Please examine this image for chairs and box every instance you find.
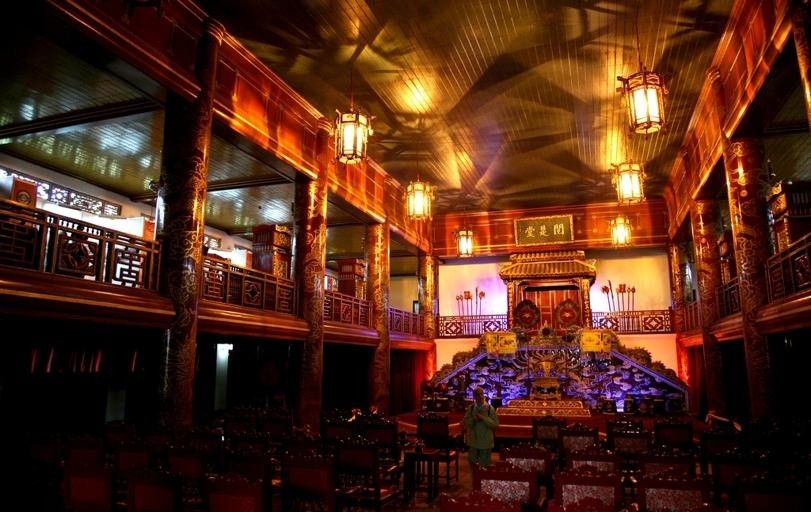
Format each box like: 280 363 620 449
72 404 805 511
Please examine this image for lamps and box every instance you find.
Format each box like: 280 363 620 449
608 1 671 249
332 62 478 260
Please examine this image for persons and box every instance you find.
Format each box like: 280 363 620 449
462 387 499 472
